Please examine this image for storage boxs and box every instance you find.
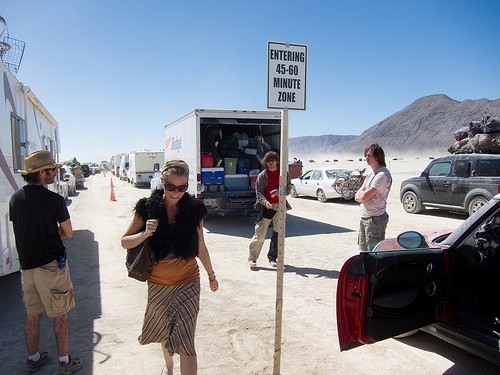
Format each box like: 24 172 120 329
249 174 257 190
201 168 224 185
224 174 249 191
245 148 257 155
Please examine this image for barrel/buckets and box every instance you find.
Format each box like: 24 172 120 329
202 152 214 168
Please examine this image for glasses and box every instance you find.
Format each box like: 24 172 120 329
164 179 188 192
44 168 56 172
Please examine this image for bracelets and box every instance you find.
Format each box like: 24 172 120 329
209 276 215 281
208 271 215 275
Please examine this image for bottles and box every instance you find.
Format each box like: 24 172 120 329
58 256 66 269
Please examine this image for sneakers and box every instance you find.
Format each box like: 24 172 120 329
24 350 50 374
56 355 83 375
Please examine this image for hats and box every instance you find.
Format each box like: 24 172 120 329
17 149 63 175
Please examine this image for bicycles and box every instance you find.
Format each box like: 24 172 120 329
334 168 367 199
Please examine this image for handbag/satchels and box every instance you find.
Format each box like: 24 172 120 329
125 198 156 282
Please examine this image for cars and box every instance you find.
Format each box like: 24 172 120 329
289 169 355 203
81 162 100 177
60 164 76 195
69 166 86 190
400 154 500 217
58 170 70 203
335 193 500 375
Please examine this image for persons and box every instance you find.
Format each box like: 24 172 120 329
248 151 292 267
355 143 392 252
120 159 218 375
8 149 84 375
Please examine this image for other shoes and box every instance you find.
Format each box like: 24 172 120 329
269 260 278 266
249 259 256 266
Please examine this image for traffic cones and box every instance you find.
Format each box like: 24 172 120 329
110 178 118 201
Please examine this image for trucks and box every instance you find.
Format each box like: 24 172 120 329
0 57 62 278
125 150 164 188
100 153 126 177
150 108 281 219
119 155 129 182
